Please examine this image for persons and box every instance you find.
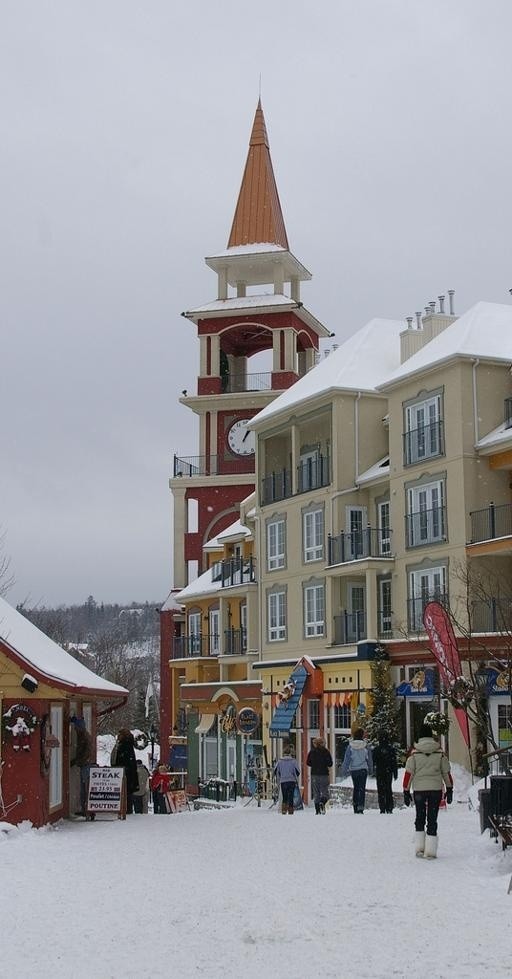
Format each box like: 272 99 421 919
338 728 373 816
402 722 455 862
67 716 97 817
372 729 399 815
305 735 334 815
405 734 447 812
272 745 301 816
108 727 139 813
132 758 176 814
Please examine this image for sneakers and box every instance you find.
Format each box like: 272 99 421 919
75 809 96 817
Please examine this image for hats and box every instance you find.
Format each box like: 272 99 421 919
74 719 85 728
119 728 130 738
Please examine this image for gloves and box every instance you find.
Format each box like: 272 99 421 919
444 788 454 804
403 790 413 807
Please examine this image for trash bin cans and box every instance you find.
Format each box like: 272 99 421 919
204 778 227 801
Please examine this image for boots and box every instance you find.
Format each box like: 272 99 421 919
413 831 438 860
281 797 394 815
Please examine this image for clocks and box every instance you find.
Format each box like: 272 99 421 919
224 416 256 459
